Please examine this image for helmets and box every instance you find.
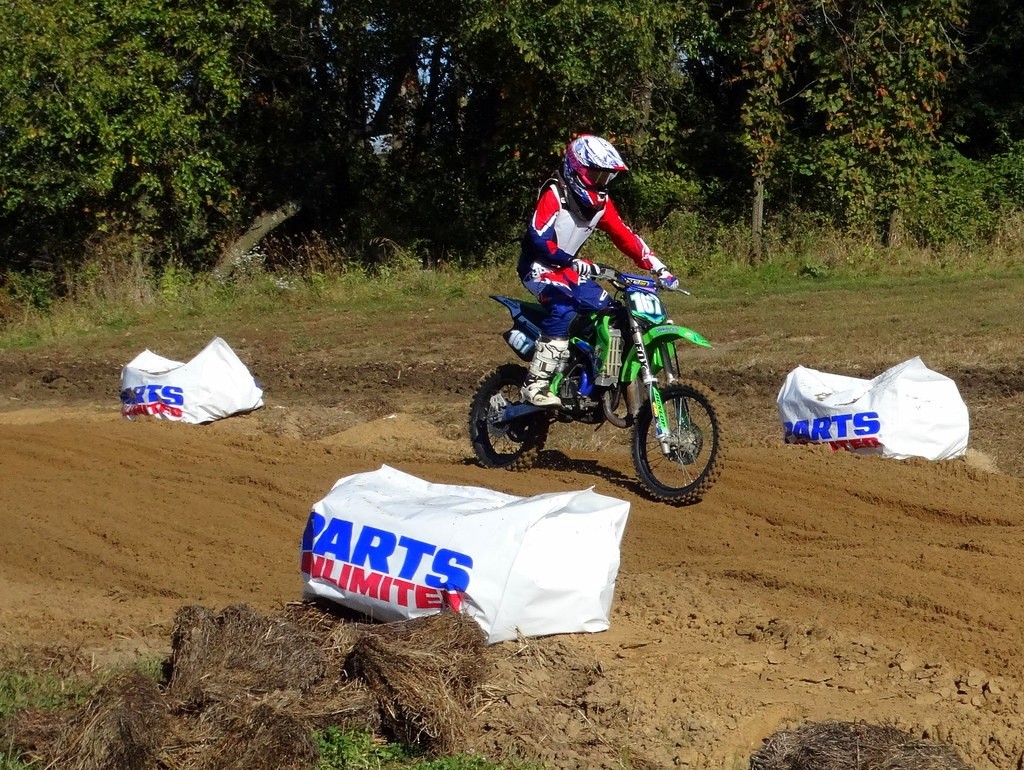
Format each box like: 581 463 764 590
559 135 629 210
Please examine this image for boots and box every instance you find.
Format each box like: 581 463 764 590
520 336 569 407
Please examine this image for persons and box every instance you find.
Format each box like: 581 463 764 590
514 136 680 407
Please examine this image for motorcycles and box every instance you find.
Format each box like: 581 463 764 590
467 262 729 506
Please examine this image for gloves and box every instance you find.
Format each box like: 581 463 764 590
572 259 600 277
657 268 679 291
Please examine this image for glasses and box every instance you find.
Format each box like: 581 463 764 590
580 166 619 189
